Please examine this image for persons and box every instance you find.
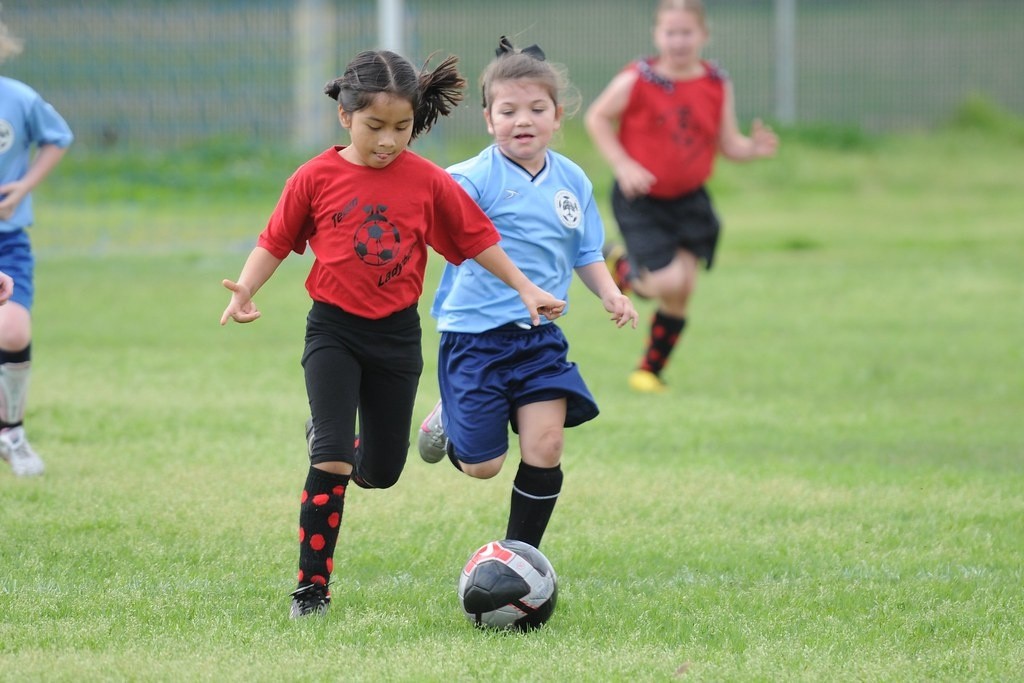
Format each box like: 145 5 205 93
0 25 73 478
419 36 639 549
583 0 779 394
220 51 566 622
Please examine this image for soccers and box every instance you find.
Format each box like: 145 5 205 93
456 538 560 637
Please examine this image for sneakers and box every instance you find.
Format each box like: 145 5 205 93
288 587 333 623
1 426 43 477
417 399 449 464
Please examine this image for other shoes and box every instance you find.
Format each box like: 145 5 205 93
627 371 675 395
601 237 627 297
